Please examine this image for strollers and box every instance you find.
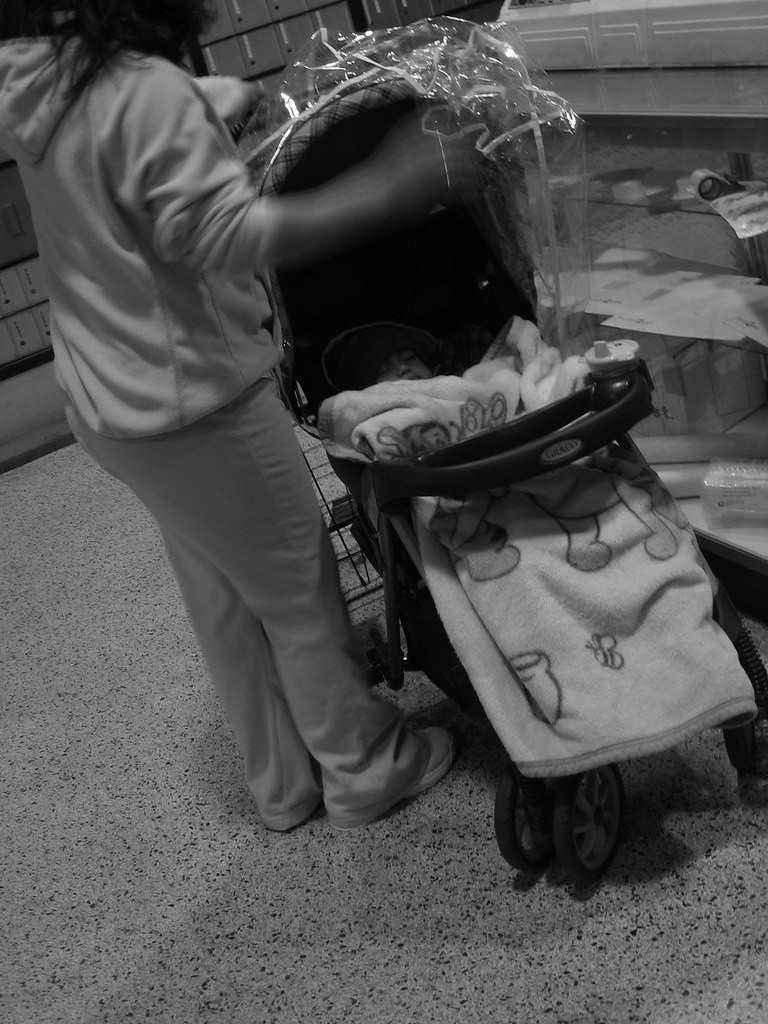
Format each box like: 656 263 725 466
246 73 768 879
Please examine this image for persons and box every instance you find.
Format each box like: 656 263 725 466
0 1 458 830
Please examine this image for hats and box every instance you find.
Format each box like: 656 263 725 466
322 317 438 392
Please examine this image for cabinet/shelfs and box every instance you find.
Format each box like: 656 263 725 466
538 171 768 631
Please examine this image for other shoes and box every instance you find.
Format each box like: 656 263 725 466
327 726 455 831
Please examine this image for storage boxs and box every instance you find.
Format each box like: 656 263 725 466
15 256 49 308
0 319 18 365
198 0 309 46
31 302 53 348
310 1 355 34
201 36 249 81
236 25 285 77
0 266 29 318
273 14 319 65
6 310 42 358
